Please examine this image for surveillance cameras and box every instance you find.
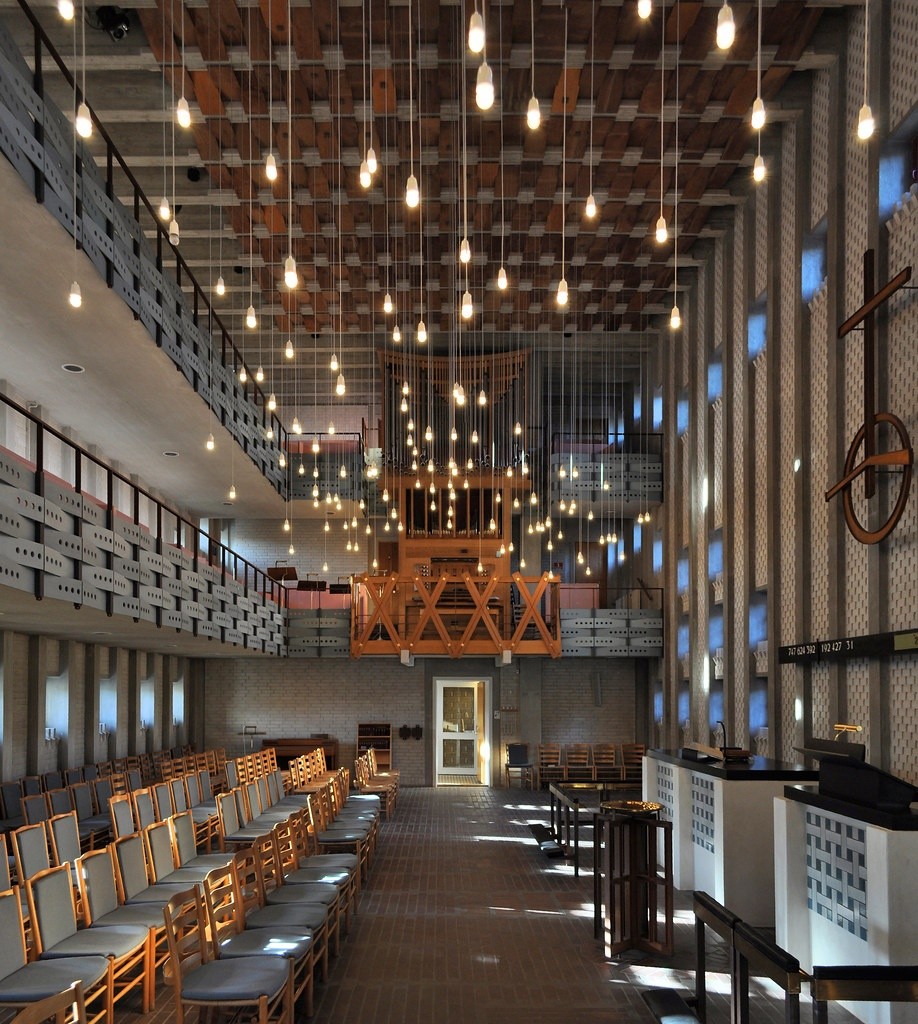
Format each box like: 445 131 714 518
95 6 127 42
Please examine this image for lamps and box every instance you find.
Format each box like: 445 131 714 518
56 0 874 580
834 724 863 742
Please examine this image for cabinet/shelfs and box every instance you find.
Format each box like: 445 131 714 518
356 722 392 771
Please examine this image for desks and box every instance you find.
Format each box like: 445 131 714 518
260 740 339 771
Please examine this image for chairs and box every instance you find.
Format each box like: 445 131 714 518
504 738 646 793
0 744 400 1024
